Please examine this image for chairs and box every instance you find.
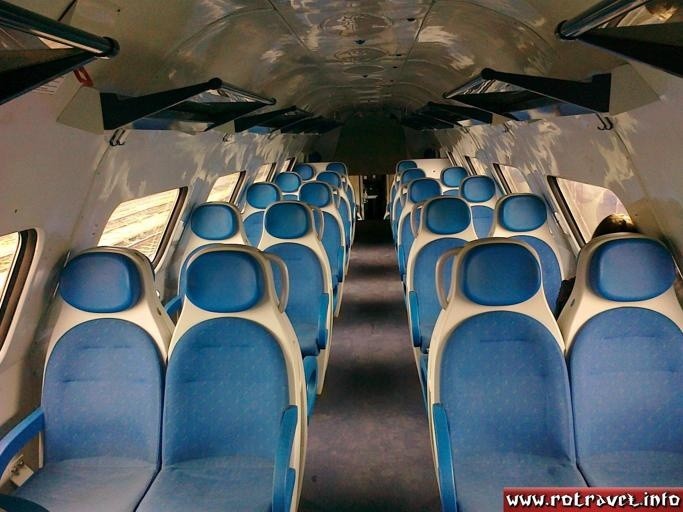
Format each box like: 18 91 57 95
0 158 683 512
